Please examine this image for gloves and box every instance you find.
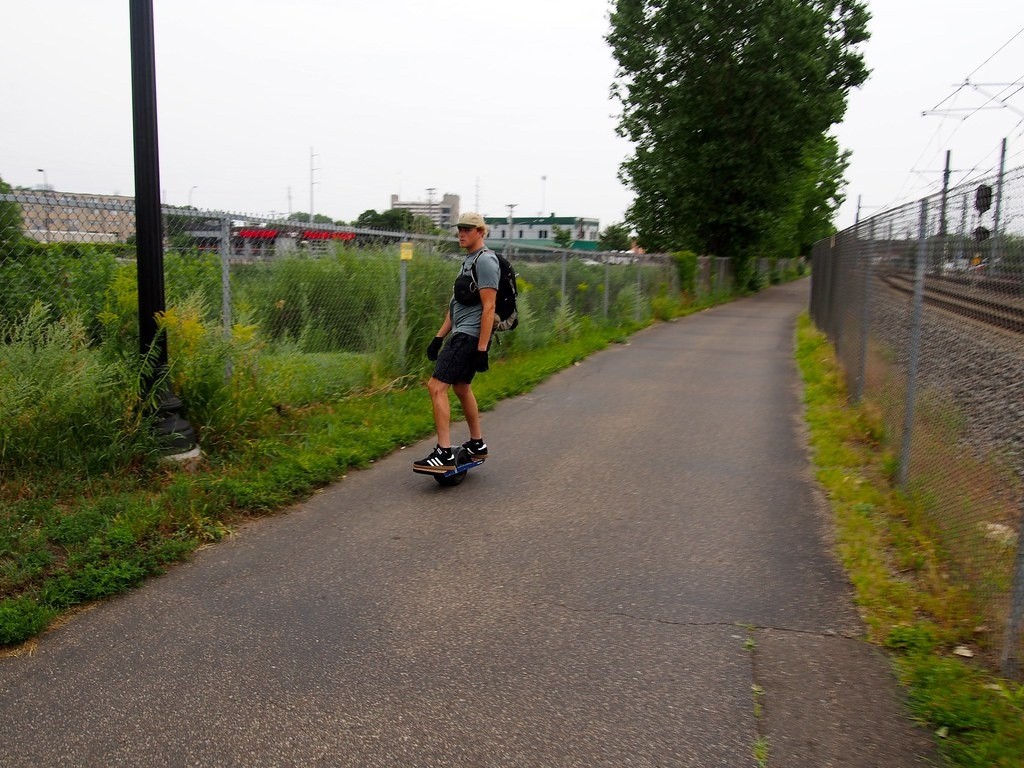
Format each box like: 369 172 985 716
472 350 489 372
427 337 443 360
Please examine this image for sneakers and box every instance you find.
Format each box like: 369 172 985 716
462 441 488 458
413 444 456 471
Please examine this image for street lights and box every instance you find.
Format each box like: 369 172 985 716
505 203 518 261
188 185 199 208
38 168 48 196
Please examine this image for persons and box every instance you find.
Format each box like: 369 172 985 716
413 212 501 470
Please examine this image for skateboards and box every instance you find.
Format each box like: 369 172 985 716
434 445 485 486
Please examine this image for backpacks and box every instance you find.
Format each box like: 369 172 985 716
470 250 519 332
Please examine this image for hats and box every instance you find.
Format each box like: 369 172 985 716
450 212 485 228
